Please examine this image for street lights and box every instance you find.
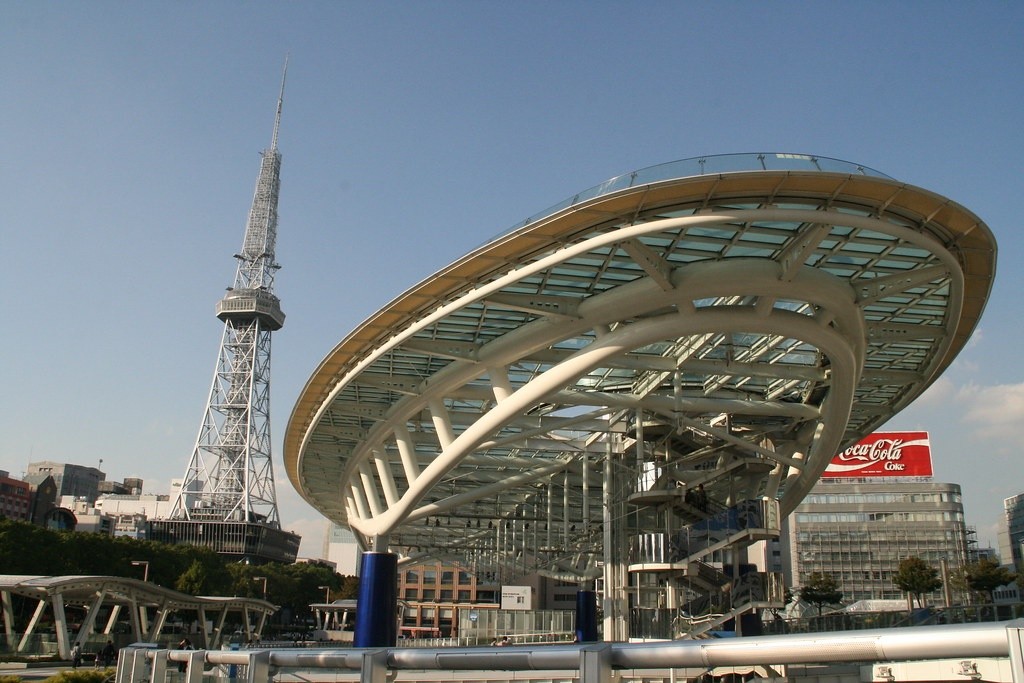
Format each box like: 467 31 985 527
318 586 329 604
254 576 266 601
131 561 149 582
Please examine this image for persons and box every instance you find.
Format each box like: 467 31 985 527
71 642 82 669
95 640 119 671
686 483 710 511
178 637 192 680
716 572 737 613
291 629 311 646
249 628 260 645
424 515 605 534
489 636 514 648
